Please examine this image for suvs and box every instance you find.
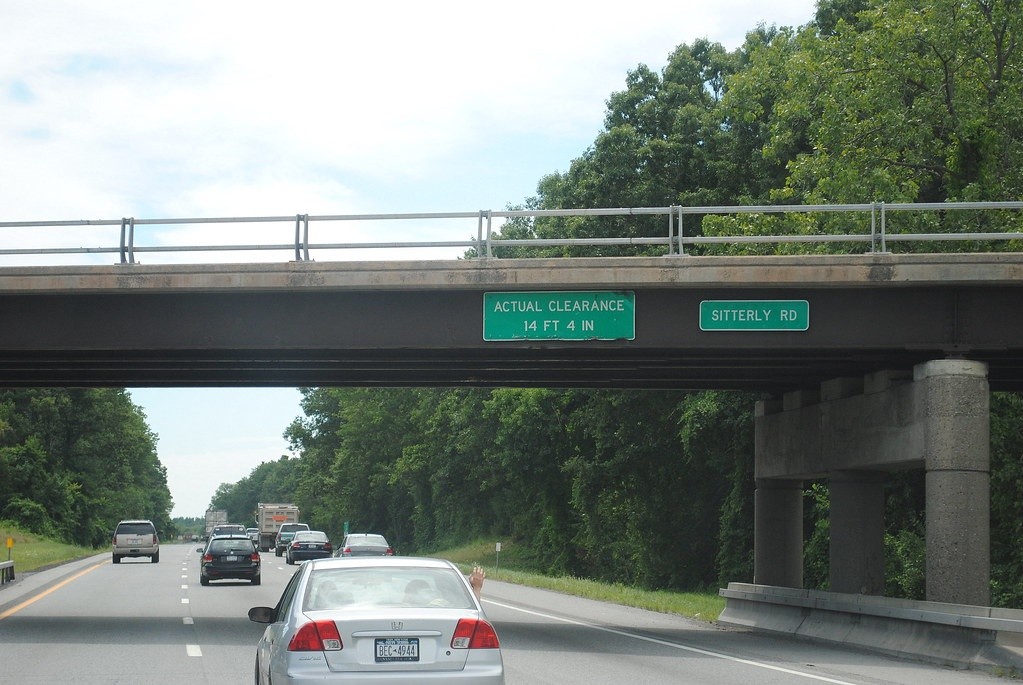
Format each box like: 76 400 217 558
196 532 262 586
112 519 160 563
202 524 254 546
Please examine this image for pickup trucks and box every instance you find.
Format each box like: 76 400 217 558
286 530 333 565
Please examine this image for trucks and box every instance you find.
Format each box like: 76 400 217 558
203 509 229 541
254 503 301 552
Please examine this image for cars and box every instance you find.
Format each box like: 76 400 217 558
249 554 506 685
334 533 395 557
246 528 259 544
271 522 312 556
